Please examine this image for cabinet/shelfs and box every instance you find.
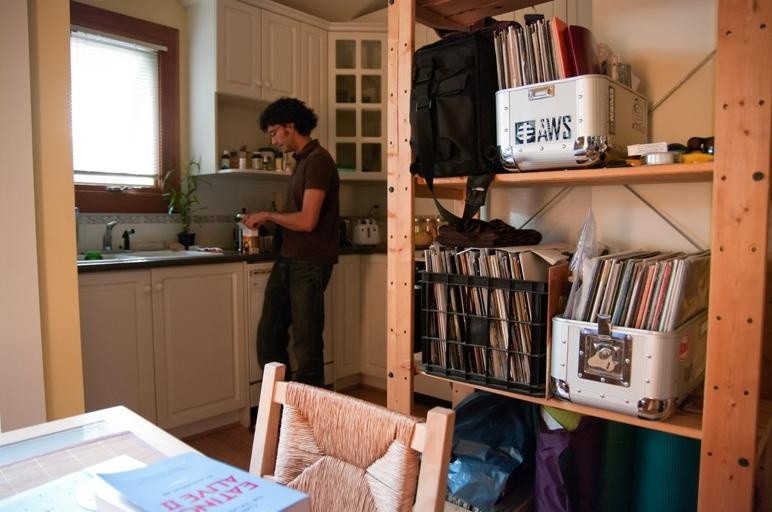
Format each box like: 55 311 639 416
214 92 293 174
216 1 300 101
329 29 387 174
76 265 244 424
387 0 772 512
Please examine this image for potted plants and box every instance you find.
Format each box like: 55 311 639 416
159 158 213 249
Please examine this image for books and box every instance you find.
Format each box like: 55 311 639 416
424 247 571 386
90 449 312 512
493 15 605 91
565 248 711 331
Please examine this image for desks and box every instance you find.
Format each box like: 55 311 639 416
0 402 212 512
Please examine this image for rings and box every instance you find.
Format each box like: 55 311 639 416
244 222 248 225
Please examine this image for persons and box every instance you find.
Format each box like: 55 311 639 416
236 94 342 439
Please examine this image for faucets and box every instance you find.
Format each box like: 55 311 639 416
122 228 135 250
103 221 118 250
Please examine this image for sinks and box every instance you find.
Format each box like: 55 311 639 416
77 253 137 264
124 250 224 258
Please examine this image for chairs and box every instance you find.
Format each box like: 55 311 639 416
243 361 457 511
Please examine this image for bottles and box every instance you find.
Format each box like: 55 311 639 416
221 146 284 171
237 208 260 257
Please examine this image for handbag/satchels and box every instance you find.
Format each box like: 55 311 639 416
407 12 523 179
